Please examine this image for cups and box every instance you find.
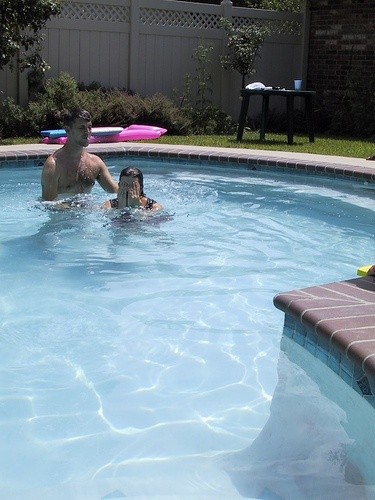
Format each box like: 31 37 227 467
294 80 302 90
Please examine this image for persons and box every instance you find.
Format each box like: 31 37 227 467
41 108 119 219
102 166 164 215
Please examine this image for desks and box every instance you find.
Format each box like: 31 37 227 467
236 89 315 146
